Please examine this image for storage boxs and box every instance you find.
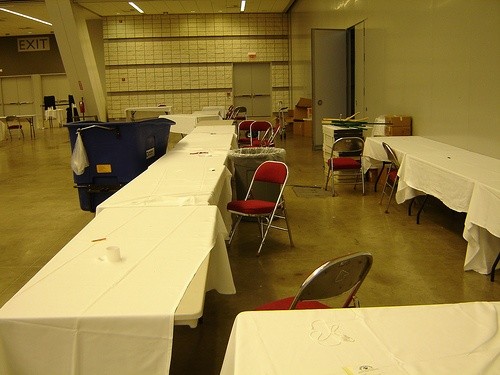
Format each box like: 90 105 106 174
373 116 413 135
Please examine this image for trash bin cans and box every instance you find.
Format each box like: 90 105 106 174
228 146 286 223
63 117 176 214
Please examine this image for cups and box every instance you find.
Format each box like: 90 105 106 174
106 246 120 262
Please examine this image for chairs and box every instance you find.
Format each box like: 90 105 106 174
230 109 240 120
254 253 373 310
6 116 25 140
227 161 295 255
250 121 274 147
234 106 247 120
379 142 426 215
225 105 234 120
325 137 366 197
237 120 255 148
261 125 281 146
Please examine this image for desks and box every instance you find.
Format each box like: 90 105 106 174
0 106 500 375
